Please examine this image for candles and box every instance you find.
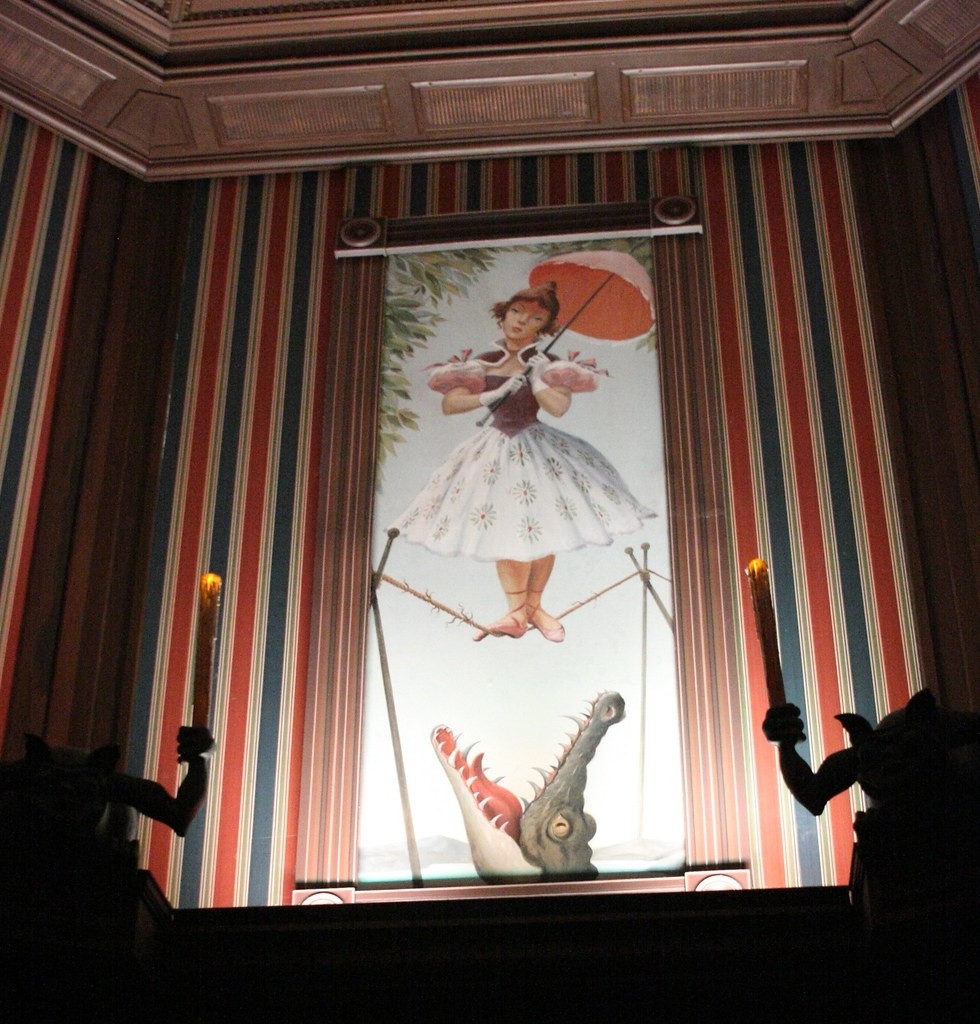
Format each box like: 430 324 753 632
192 573 222 728
745 559 788 707
